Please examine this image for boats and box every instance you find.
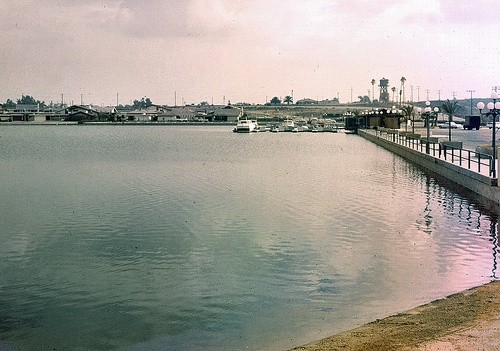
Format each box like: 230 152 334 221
232 116 340 133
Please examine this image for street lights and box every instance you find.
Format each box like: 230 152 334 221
416 100 439 154
400 76 406 117
476 91 500 178
391 87 396 113
371 78 376 111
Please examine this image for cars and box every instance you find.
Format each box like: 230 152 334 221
485 121 500 129
438 121 458 129
